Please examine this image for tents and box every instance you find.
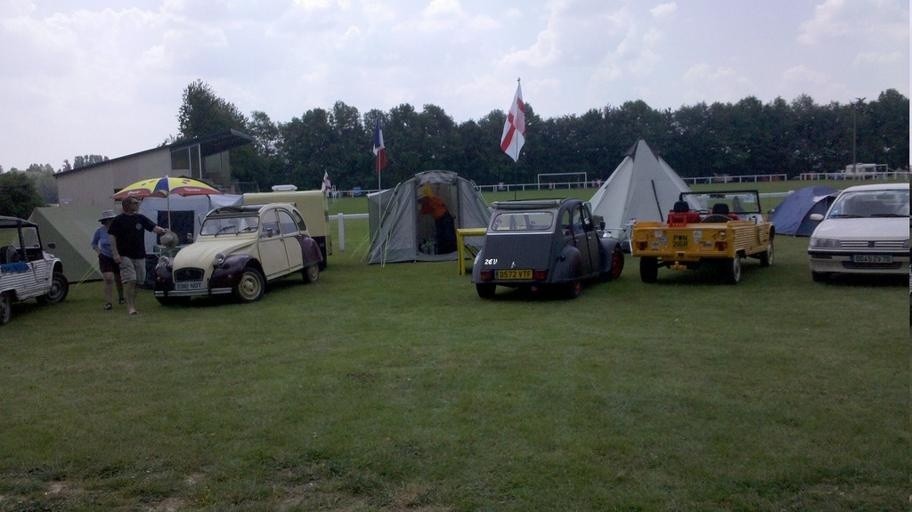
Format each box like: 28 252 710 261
767 184 843 237
18 206 105 283
572 136 704 254
366 170 492 265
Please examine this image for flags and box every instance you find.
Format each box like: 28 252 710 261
500 82 526 162
373 119 389 172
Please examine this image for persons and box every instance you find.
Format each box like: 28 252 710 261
90 210 126 311
108 196 168 315
416 197 457 254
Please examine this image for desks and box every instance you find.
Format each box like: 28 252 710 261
455 228 486 276
144 251 177 289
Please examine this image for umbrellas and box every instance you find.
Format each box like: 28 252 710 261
110 175 222 230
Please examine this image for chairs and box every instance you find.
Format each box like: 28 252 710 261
702 214 732 224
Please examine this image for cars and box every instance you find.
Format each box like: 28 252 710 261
152 199 325 311
704 173 735 185
350 186 363 199
805 181 912 290
467 196 628 304
755 162 910 183
496 182 507 192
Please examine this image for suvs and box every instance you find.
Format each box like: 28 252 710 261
630 182 779 289
1 213 71 331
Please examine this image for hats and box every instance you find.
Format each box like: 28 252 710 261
96 210 118 222
